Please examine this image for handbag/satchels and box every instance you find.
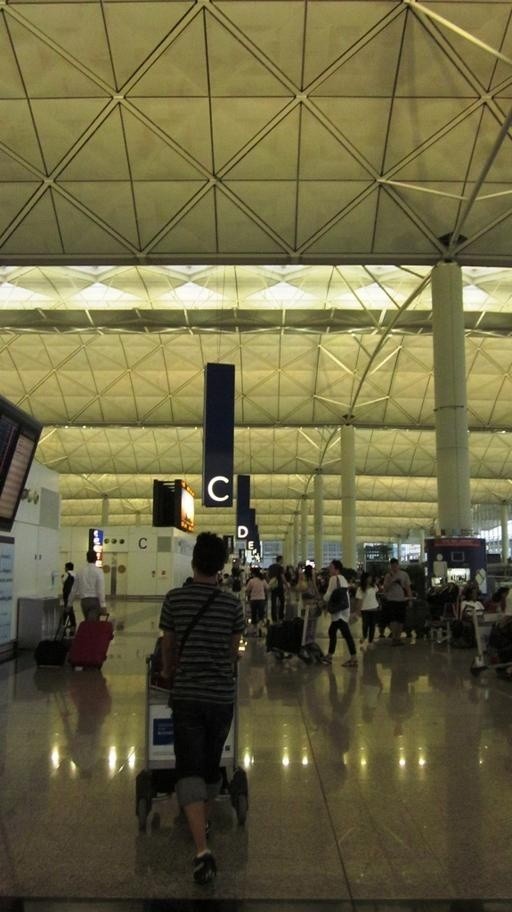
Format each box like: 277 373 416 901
326 587 349 613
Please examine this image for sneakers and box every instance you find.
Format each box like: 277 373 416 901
192 851 218 885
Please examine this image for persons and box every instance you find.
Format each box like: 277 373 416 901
65 549 107 618
159 530 245 885
61 562 77 636
231 553 512 680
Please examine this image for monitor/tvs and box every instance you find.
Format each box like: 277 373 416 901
448 568 470 584
0 395 44 532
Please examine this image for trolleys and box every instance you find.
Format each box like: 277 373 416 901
136 637 249 830
266 603 324 663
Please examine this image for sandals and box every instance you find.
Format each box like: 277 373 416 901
318 656 333 664
341 659 358 668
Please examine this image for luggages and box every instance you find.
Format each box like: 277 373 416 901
34 612 69 668
67 613 114 671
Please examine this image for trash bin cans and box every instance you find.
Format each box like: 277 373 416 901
17 596 60 651
